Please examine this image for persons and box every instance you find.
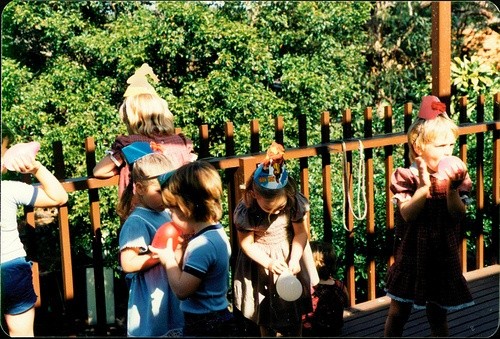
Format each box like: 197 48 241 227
381 115 477 338
92 91 199 218
147 158 231 338
1 122 69 337
120 151 185 338
230 162 321 338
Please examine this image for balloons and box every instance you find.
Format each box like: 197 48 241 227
275 271 303 302
151 221 181 251
438 156 466 179
